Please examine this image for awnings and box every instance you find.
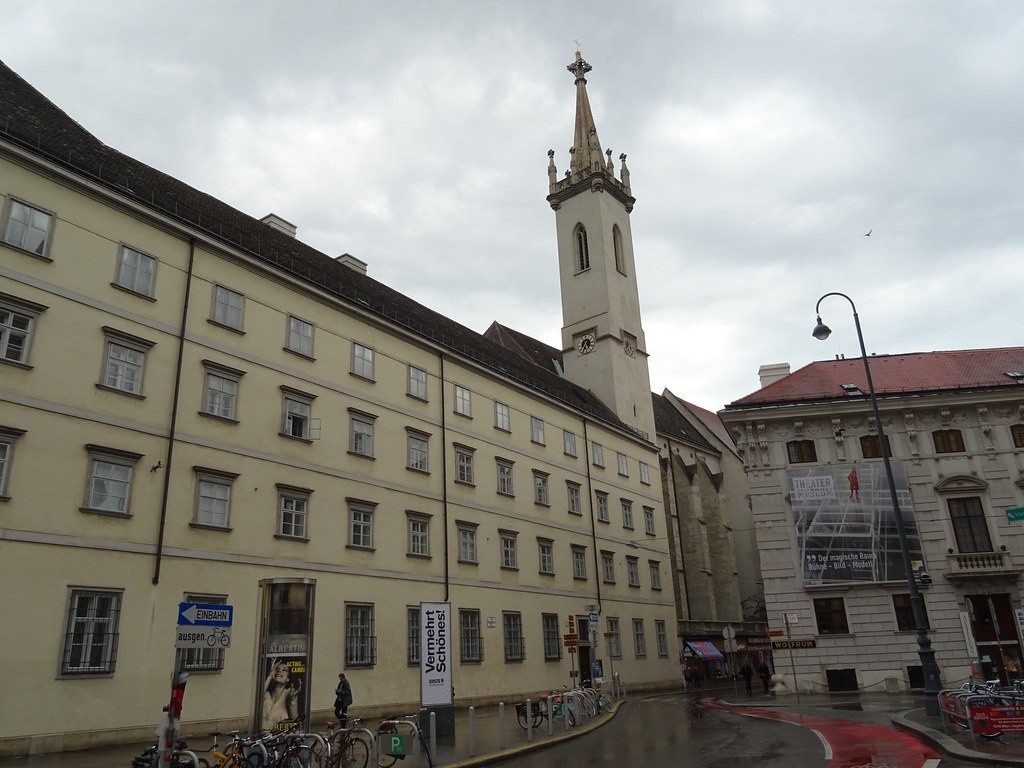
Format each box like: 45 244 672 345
685 639 724 661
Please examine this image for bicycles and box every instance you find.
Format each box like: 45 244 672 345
514 678 619 729
369 706 433 768
128 705 370 768
948 678 1024 739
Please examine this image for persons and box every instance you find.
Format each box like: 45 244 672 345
334 673 353 730
759 661 770 695
684 665 701 689
738 662 756 695
261 657 302 731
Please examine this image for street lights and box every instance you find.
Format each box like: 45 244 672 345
809 292 948 716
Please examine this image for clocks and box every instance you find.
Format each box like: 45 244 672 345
622 336 634 355
577 334 595 355
